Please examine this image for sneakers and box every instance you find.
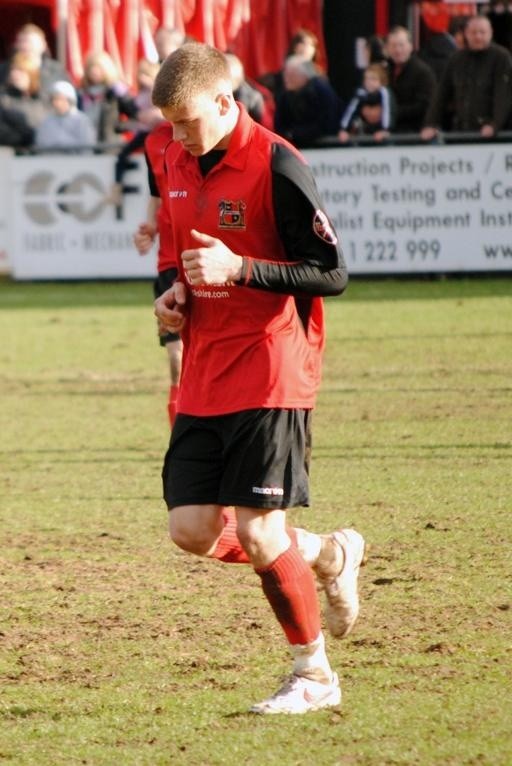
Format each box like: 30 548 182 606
249 673 342 716
325 525 366 640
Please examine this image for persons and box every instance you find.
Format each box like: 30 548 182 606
153 44 363 714
438 13 512 145
133 120 183 429
220 29 439 149
0 24 165 149
